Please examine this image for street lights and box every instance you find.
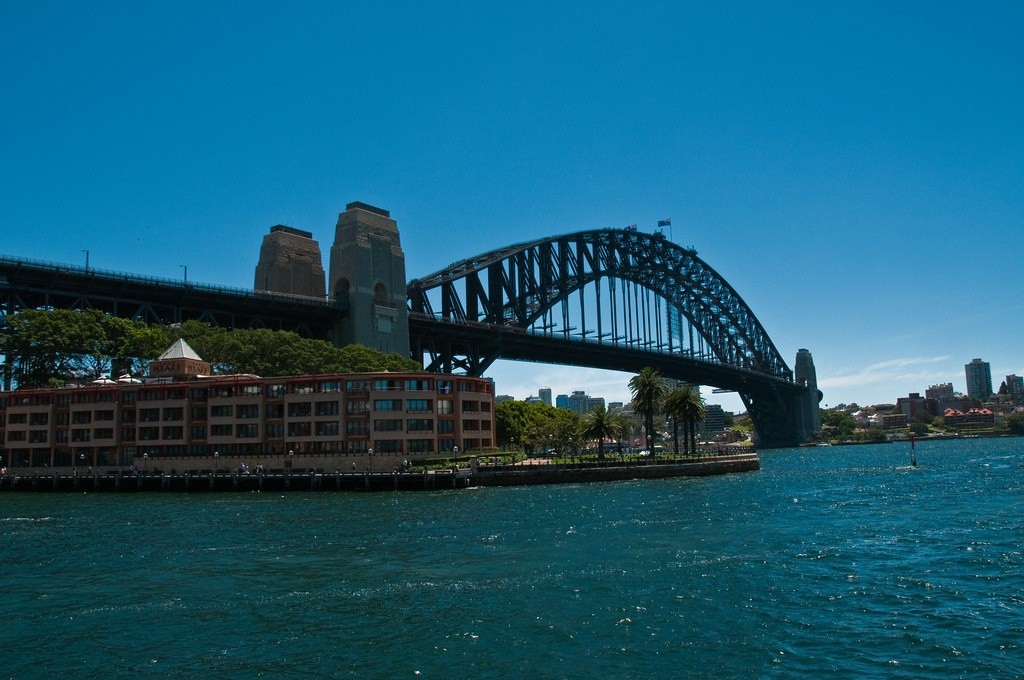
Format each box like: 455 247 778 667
453 445 459 466
214 451 219 474
697 434 701 454
80 454 84 476
511 436 516 472
143 453 148 474
368 448 373 473
179 264 187 282
289 450 293 475
82 249 90 273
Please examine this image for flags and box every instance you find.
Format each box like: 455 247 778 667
658 218 670 226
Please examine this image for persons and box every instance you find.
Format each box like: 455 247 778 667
402 458 412 472
129 464 138 476
352 460 357 472
0 466 8 477
241 463 263 476
74 466 93 477
